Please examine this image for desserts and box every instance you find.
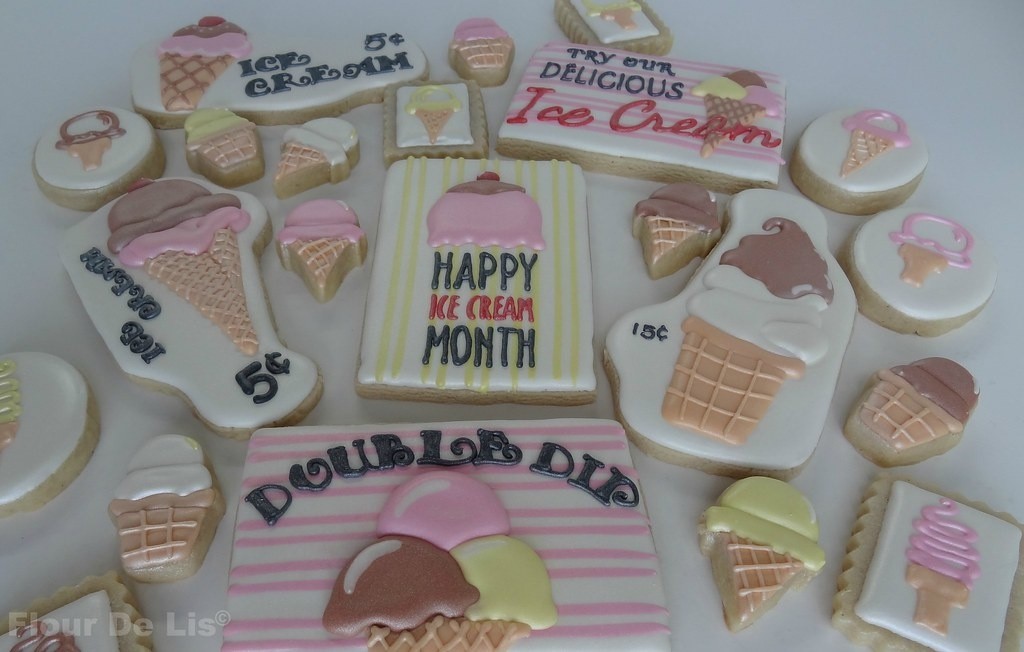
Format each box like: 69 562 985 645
553 0 673 56
184 108 264 187
274 117 359 198
60 178 324 440
275 199 367 304
497 42 787 193
221 418 673 652
603 187 858 482
790 107 929 214
842 209 997 336
32 106 166 212
106 433 225 582
696 476 825 634
0 352 100 518
449 17 515 86
383 80 488 165
0 572 153 652
843 354 978 467
355 155 597 405
632 181 721 278
130 16 430 131
832 468 1024 652
